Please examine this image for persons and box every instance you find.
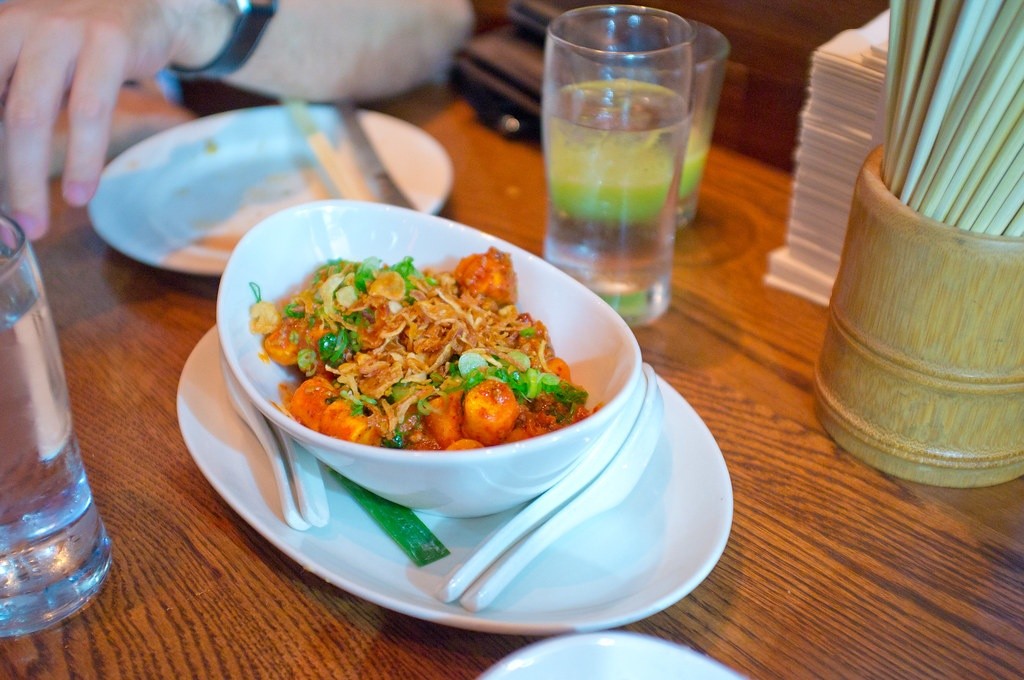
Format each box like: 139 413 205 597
0 0 476 250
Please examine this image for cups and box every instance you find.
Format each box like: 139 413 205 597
540 5 731 332
0 211 111 639
812 144 1024 488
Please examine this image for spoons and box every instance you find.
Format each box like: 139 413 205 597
219 341 329 530
439 361 663 612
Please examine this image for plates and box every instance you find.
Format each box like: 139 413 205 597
176 318 734 680
89 99 455 277
473 630 745 680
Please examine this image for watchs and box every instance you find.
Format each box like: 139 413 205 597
170 0 279 79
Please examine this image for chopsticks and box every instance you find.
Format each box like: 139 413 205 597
884 0 1024 236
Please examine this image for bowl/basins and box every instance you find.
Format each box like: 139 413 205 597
217 199 643 518
